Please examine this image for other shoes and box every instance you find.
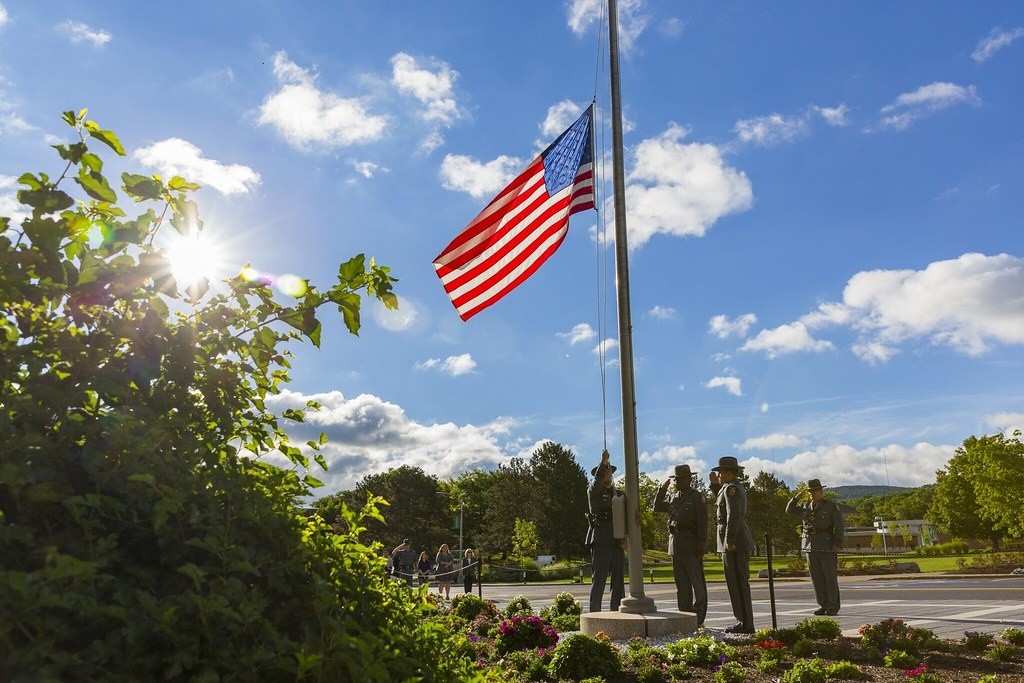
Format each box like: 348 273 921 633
826 609 838 615
814 607 826 615
725 623 755 634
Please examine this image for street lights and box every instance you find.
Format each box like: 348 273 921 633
435 490 464 561
875 516 887 556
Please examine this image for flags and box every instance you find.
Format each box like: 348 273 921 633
432 99 598 322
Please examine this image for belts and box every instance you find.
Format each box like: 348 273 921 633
670 523 697 531
803 528 831 535
716 517 725 524
401 564 414 570
590 512 611 521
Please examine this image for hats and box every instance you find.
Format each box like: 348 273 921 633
403 539 412 544
669 464 697 477
806 478 827 491
711 456 745 471
591 462 618 476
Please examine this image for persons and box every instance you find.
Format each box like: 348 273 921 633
654 465 709 627
584 449 628 612
369 539 417 587
417 551 431 585
785 480 844 616
436 544 454 600
462 549 477 594
709 456 755 633
